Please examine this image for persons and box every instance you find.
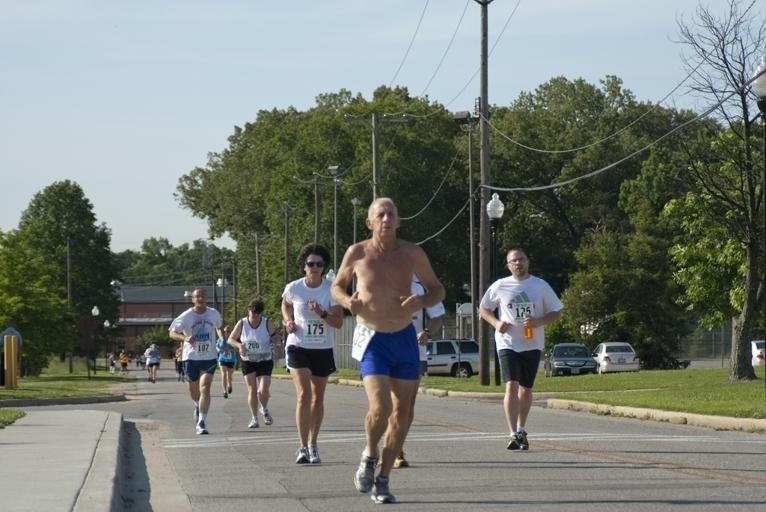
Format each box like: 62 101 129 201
168 288 225 435
109 342 184 382
281 243 343 463
0 325 23 386
329 198 447 504
479 247 564 449
228 298 283 428
377 274 446 468
216 324 237 398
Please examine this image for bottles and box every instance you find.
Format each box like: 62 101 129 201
522 311 534 341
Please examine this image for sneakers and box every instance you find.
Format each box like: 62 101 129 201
259 408 273 426
393 451 410 468
354 445 380 493
307 442 319 464
370 475 396 506
295 445 309 464
223 385 233 399
507 430 530 451
247 416 259 428
193 401 209 435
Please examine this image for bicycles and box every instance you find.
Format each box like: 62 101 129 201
639 353 691 370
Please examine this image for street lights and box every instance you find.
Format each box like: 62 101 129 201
349 195 360 294
215 277 228 324
486 191 507 386
103 319 110 371
90 306 101 375
109 278 123 361
184 289 191 308
450 107 493 341
328 161 340 275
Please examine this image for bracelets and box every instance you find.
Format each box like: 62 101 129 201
424 328 432 337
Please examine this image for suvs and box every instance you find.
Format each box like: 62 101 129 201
423 337 481 378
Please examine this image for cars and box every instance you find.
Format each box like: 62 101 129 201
592 342 639 373
543 343 596 377
750 340 766 366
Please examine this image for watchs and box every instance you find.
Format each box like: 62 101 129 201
321 309 328 318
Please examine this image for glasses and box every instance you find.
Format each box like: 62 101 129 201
304 261 324 268
